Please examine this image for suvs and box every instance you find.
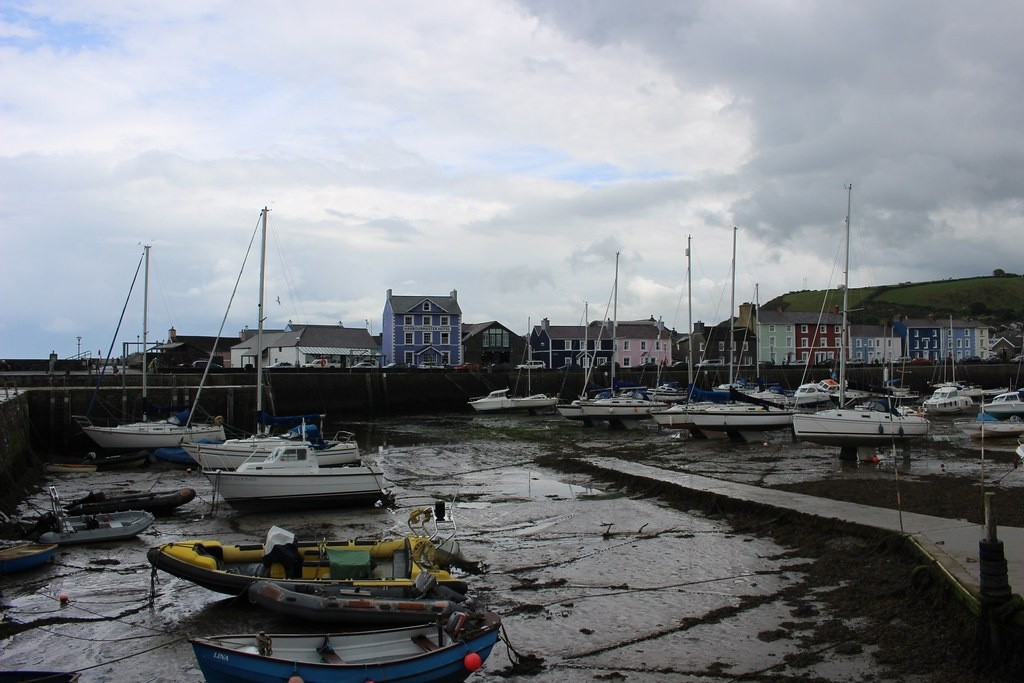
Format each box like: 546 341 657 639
514 360 547 370
892 357 911 363
847 357 865 365
418 362 445 369
193 361 223 369
695 359 723 368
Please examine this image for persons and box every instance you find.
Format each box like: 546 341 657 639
772 358 886 368
319 358 448 371
46 355 123 376
149 357 157 373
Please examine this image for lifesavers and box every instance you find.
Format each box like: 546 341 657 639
215 416 224 426
410 507 431 524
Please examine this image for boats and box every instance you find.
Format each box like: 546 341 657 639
147 497 501 683
923 314 1024 440
0 670 83 683
0 484 197 575
196 413 396 509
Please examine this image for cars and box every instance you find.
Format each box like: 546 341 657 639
453 362 479 370
381 362 408 369
556 364 580 371
758 360 775 368
345 362 380 369
264 361 296 368
640 363 656 368
1011 356 1024 363
912 358 930 365
788 360 806 366
818 358 836 367
959 355 981 363
669 361 687 368
594 361 620 368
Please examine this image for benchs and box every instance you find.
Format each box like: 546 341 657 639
315 635 346 664
109 521 123 528
411 634 440 652
62 521 75 533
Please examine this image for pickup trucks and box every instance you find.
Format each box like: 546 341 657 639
301 358 341 369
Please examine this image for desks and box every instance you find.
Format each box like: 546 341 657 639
340 588 371 596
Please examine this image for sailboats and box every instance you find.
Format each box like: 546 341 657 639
556 234 921 426
790 185 929 443
176 205 361 473
72 241 228 449
466 314 559 414
682 226 801 431
